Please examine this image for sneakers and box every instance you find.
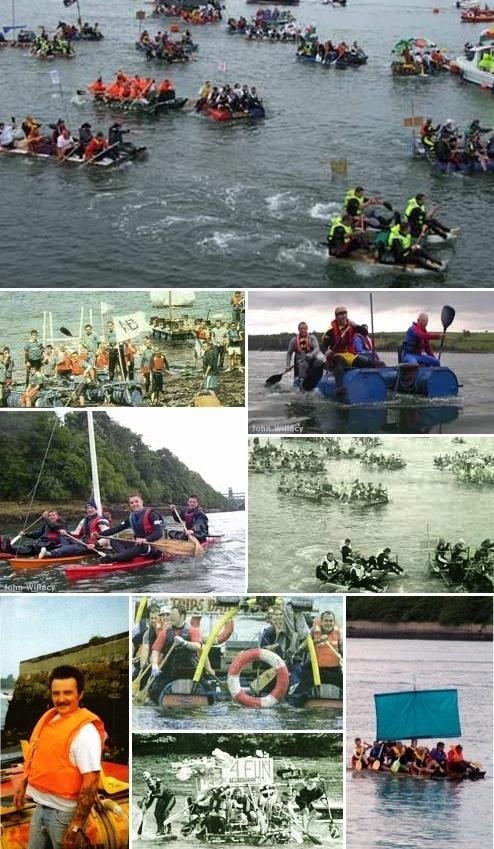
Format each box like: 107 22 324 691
0 535 5 552
39 547 47 558
5 537 12 552
335 387 345 398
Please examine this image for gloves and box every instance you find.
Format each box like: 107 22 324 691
149 667 162 679
174 636 187 647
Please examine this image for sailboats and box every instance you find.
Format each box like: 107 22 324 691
149 289 214 347
6 410 113 573
353 685 487 780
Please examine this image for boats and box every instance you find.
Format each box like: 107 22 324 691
420 138 494 175
328 219 462 276
285 405 460 432
179 750 305 846
294 363 465 404
0 552 29 558
94 90 190 114
8 365 143 407
0 746 129 849
63 535 221 581
132 597 343 713
198 104 265 121
248 437 494 593
0 139 149 168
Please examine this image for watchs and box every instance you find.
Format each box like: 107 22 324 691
70 824 82 833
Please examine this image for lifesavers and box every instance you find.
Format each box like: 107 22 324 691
227 648 288 708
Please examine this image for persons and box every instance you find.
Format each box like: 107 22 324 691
132 602 343 706
352 736 481 775
388 1 494 178
139 770 330 838
247 437 494 512
283 303 447 387
11 664 108 849
312 534 494 594
0 290 245 407
0 0 371 166
91 495 168 564
38 495 111 559
329 182 455 280
168 494 209 542
0 509 68 555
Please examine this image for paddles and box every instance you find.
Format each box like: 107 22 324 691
323 785 340 839
391 745 404 772
96 535 194 556
282 810 322 845
163 795 206 826
372 744 383 770
417 206 439 245
303 326 350 390
172 507 200 555
266 359 304 383
356 741 364 771
60 327 83 347
137 791 147 835
438 306 454 362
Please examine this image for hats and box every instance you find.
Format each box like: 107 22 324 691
157 606 171 615
85 498 96 509
335 306 346 316
